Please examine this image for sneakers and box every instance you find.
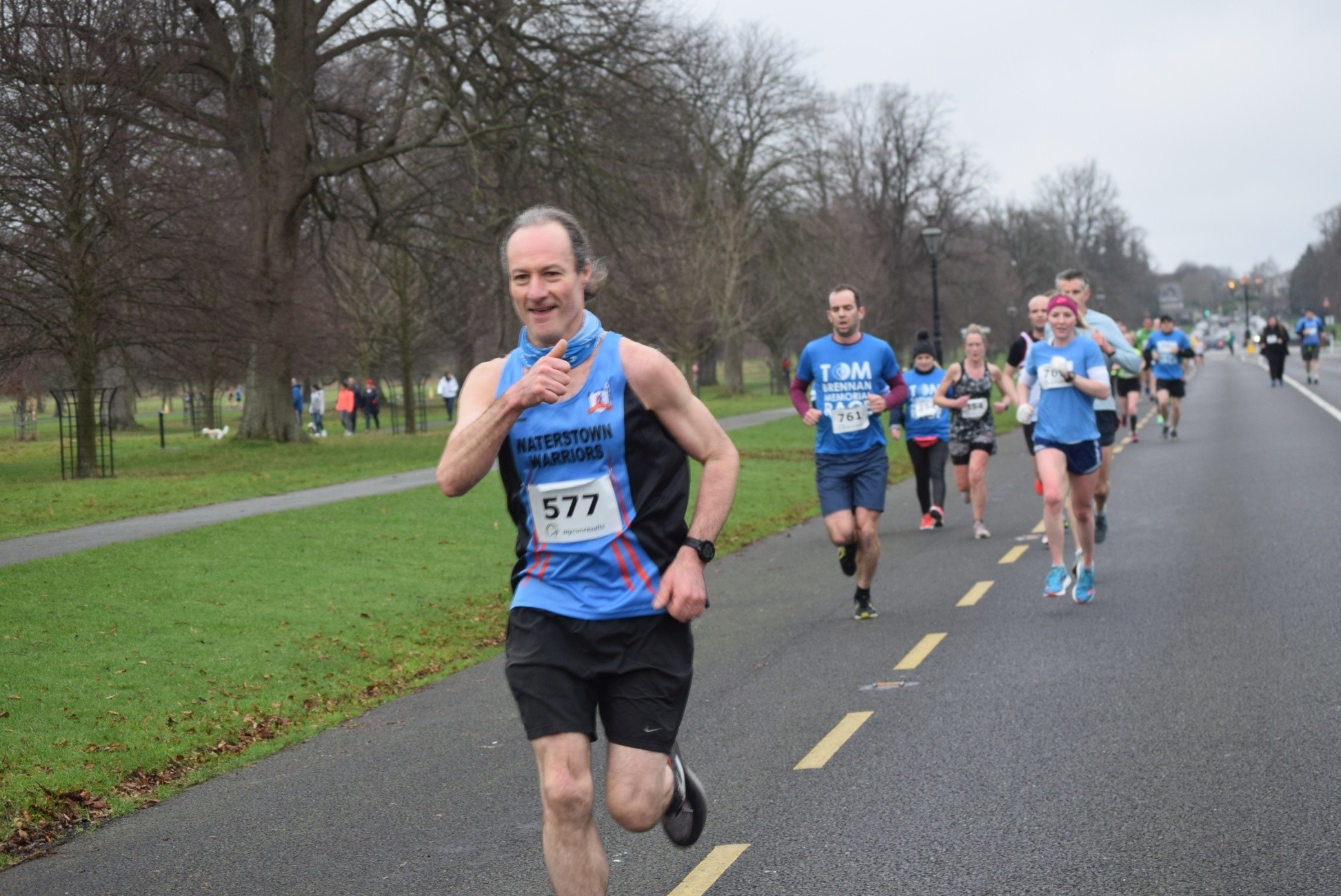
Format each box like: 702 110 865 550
973 521 991 539
1071 560 1096 602
662 741 708 848
920 514 935 530
837 541 858 577
1043 564 1071 597
930 505 944 527
963 491 971 502
853 593 877 619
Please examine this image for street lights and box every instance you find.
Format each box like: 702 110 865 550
1228 276 1265 343
1005 301 1018 344
921 215 943 370
1097 287 1105 313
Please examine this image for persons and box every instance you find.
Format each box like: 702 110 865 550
1295 309 1326 384
336 377 360 435
291 377 303 426
933 324 1011 539
437 371 459 421
889 330 953 530
783 356 790 386
692 362 699 382
308 384 327 437
360 380 380 432
791 284 909 620
1015 293 1111 603
1002 295 1069 529
1260 315 1289 387
1111 313 1204 443
227 385 242 410
1044 268 1142 577
1226 330 1234 354
436 205 741 896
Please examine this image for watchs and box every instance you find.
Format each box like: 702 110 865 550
1066 372 1075 382
682 536 715 562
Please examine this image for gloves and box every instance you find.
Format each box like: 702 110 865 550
1049 356 1074 376
1017 405 1035 424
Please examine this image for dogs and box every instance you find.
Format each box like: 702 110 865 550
202 426 229 440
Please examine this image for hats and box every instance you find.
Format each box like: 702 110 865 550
912 330 935 358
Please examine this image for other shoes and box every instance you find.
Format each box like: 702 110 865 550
1170 430 1178 438
1314 379 1318 384
1132 435 1137 442
1308 378 1311 383
1094 514 1107 543
1271 381 1276 386
1036 479 1044 495
1163 427 1169 433
1279 380 1283 386
